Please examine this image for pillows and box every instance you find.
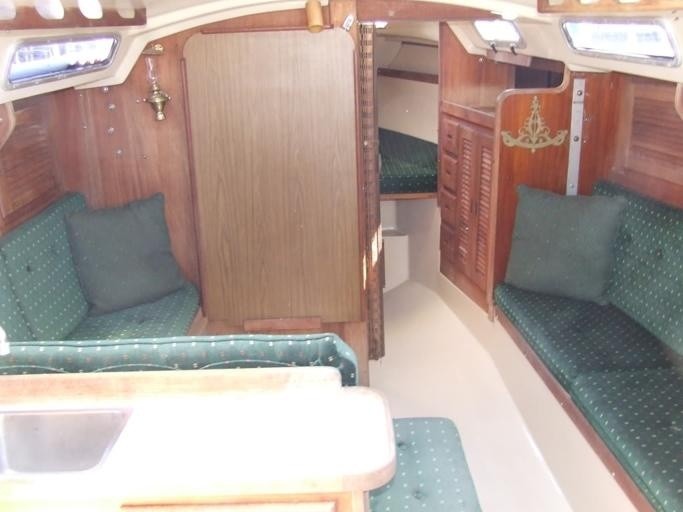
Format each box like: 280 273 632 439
58 191 190 317
502 182 628 308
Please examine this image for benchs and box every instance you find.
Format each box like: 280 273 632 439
0 191 359 382
490 178 682 512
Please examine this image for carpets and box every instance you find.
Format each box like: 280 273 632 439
369 413 483 512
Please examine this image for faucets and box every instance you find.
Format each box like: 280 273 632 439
0 325 11 357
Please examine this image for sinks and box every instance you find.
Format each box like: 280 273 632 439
1 403 138 476
